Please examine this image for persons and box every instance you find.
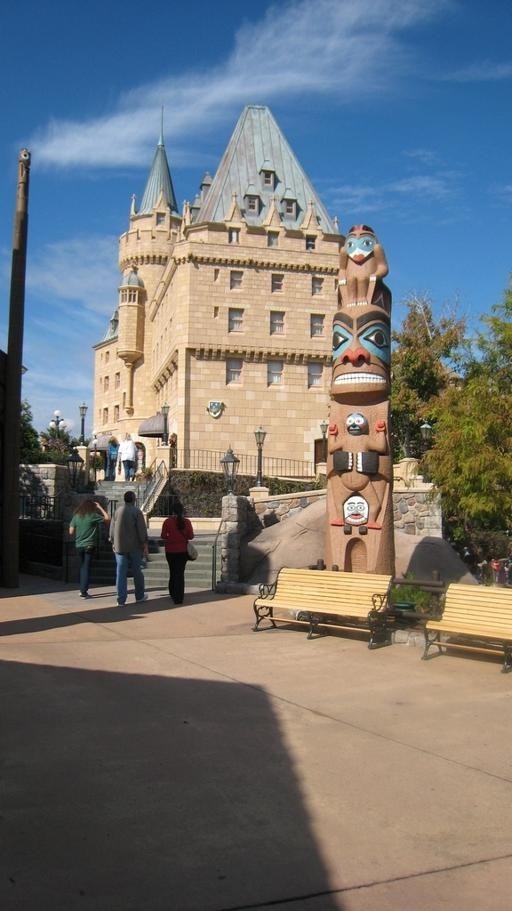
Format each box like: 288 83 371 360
104 436 120 481
457 544 512 586
108 490 149 607
118 432 138 482
160 503 194 604
68 498 111 599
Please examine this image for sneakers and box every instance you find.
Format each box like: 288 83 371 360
117 601 126 607
79 592 90 599
136 594 148 603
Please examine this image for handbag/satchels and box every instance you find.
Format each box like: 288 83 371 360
187 542 198 561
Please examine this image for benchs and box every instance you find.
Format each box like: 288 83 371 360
253 566 512 673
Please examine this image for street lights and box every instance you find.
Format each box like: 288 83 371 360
92 430 98 483
79 402 89 446
48 410 68 438
320 420 329 461
419 420 433 483
254 423 267 487
